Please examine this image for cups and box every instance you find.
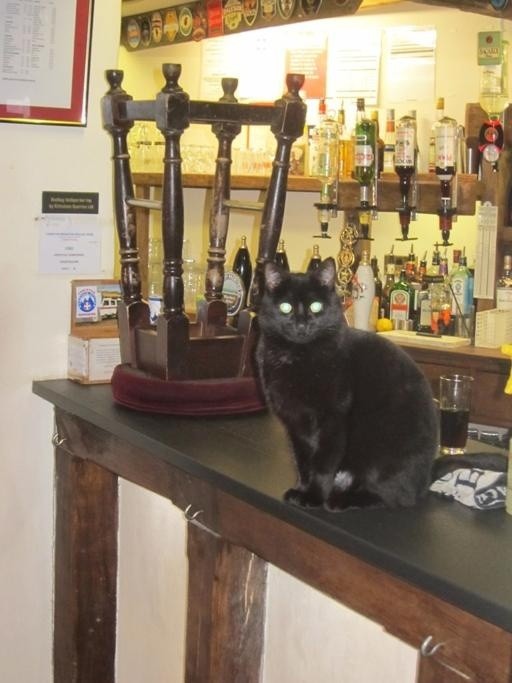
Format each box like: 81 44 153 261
438 373 476 456
455 314 475 338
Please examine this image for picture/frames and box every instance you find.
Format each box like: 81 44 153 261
0 0 95 126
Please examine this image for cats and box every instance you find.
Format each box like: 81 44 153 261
253 255 509 514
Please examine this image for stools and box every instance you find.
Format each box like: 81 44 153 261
102 60 307 420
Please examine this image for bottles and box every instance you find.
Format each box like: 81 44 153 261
309 93 460 209
479 38 512 124
351 249 475 331
495 250 512 311
